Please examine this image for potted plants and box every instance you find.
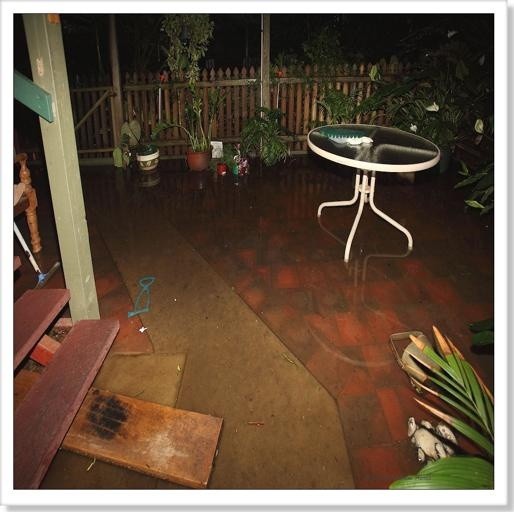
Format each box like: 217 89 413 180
386 84 463 177
110 113 161 171
240 104 294 181
151 85 234 175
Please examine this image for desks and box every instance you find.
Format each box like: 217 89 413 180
306 123 442 265
